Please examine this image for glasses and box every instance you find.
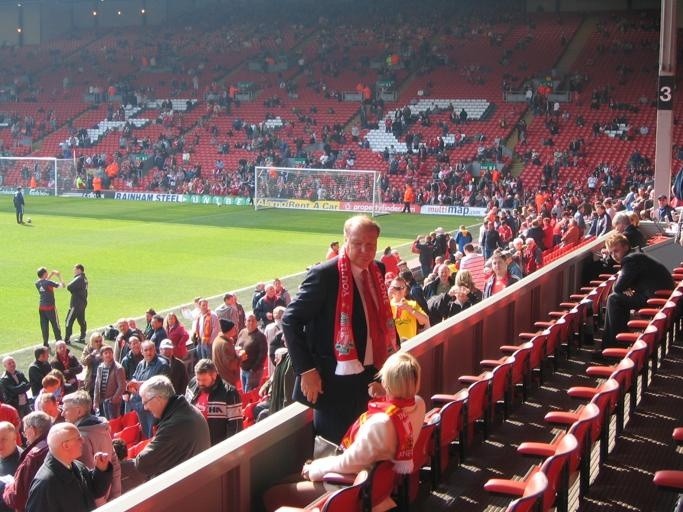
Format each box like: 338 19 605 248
141 396 155 408
21 427 32 438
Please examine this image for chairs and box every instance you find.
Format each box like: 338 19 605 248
0 9 683 512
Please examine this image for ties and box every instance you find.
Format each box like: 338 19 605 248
361 269 387 371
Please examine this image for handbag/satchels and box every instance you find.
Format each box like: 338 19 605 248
314 435 342 460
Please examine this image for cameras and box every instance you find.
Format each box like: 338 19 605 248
392 285 404 292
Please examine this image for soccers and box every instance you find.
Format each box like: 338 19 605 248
26 218 32 223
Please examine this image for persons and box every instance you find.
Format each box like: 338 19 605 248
0 16 683 512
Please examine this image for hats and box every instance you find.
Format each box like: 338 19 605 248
159 339 174 349
220 319 234 333
